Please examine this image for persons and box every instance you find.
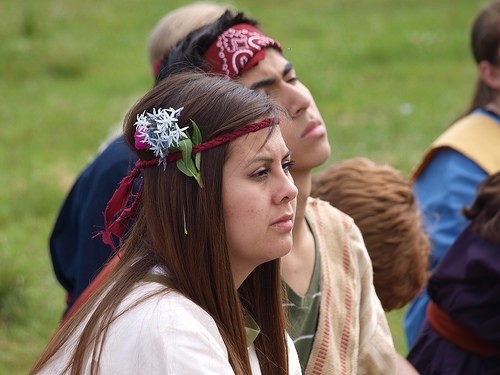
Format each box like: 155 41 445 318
311 157 430 310
49 4 235 317
403 0 500 351
157 10 419 375
407 173 500 375
31 72 302 375
